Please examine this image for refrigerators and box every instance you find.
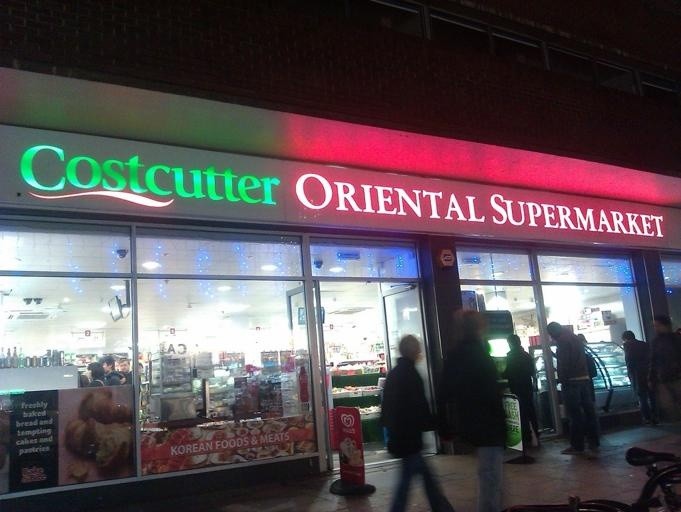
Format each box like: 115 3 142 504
529 342 639 427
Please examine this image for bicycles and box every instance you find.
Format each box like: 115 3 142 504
503 447 681 511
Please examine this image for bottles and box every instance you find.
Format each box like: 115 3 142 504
1 345 25 368
299 367 309 403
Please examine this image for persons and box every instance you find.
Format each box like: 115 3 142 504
647 314 681 428
622 330 660 427
438 307 508 511
546 321 602 454
502 333 542 454
382 334 456 511
68 356 144 387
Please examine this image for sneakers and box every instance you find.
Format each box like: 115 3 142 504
560 447 584 455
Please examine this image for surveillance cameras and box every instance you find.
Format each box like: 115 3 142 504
0 288 12 296
313 260 323 270
33 298 43 305
116 249 128 259
23 297 32 305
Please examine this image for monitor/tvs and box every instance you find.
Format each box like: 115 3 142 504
107 295 122 321
297 306 325 325
487 337 511 358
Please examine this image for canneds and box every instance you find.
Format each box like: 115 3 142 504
26 356 50 367
